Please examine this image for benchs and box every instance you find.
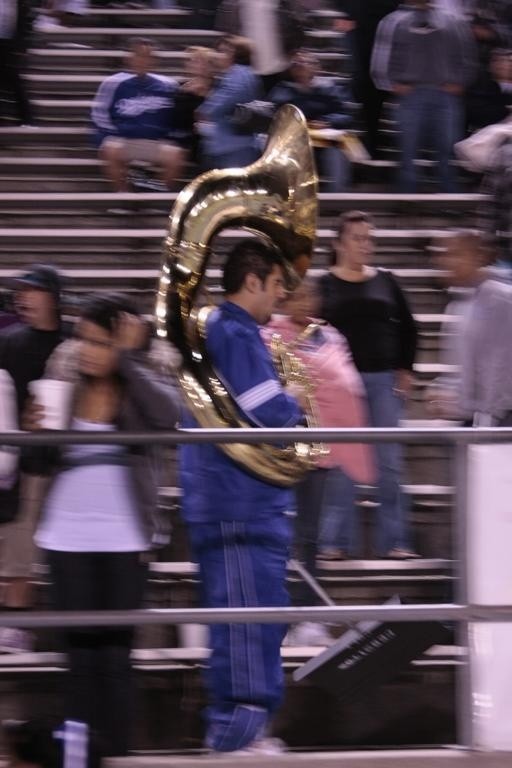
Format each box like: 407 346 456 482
0 2 511 687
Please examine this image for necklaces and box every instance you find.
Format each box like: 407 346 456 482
75 394 111 425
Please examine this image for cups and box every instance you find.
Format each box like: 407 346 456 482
177 623 210 648
25 378 74 433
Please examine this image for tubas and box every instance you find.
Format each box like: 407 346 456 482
155 103 330 489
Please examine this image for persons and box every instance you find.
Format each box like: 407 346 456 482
432 228 511 446
300 211 425 559
21 286 182 759
261 280 369 563
174 234 313 757
20 290 176 592
1 257 74 588
1 0 512 218
